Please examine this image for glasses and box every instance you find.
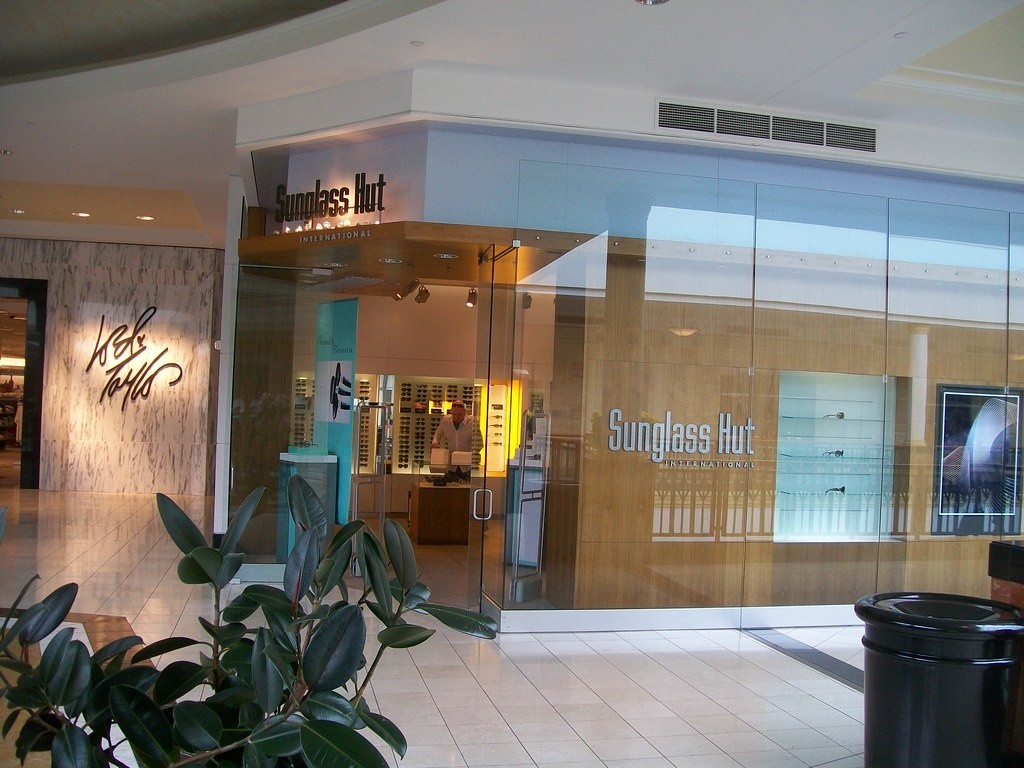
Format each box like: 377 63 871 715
292 375 504 469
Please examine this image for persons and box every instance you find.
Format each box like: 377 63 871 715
430 400 483 477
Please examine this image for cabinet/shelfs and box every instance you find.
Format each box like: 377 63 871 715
352 470 509 518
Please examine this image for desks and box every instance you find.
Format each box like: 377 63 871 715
276 454 339 563
411 481 470 545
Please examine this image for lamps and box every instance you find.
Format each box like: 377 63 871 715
465 288 478 308
396 279 420 299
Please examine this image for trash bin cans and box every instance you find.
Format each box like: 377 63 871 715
853 588 1024 768
985 537 1024 768
